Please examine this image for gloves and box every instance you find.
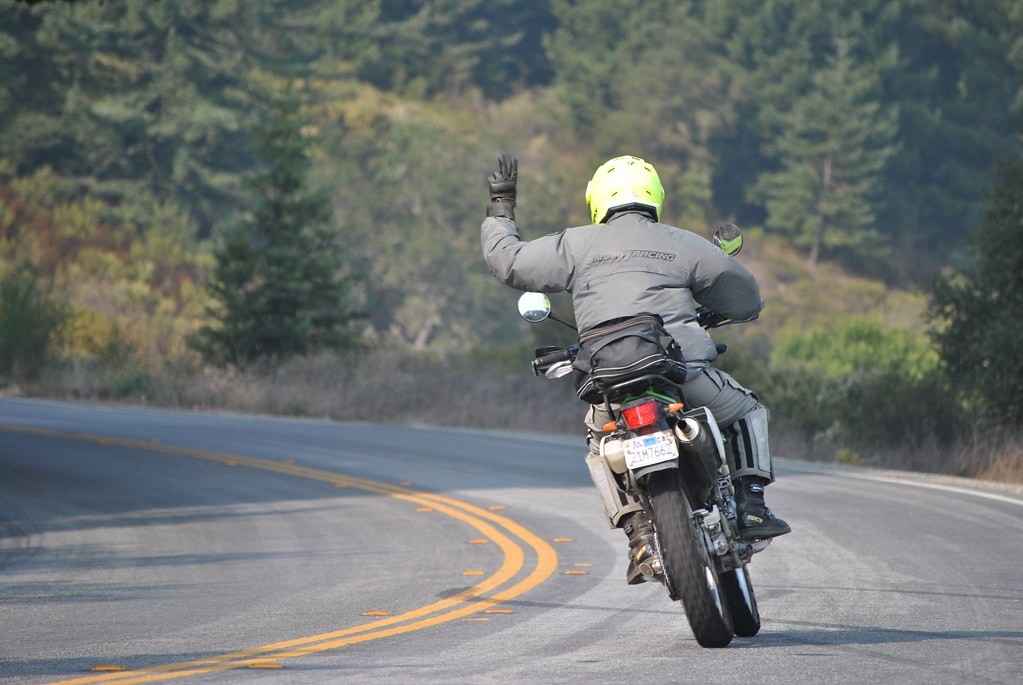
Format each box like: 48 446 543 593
486 152 518 220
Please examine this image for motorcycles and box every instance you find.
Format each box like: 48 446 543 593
517 223 775 647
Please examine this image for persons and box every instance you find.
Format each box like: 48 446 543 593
480 153 792 584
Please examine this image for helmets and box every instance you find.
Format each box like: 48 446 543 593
585 155 664 224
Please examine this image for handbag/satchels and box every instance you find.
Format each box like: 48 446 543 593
571 312 688 405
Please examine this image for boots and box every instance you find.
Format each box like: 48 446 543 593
736 496 791 539
626 523 654 585
